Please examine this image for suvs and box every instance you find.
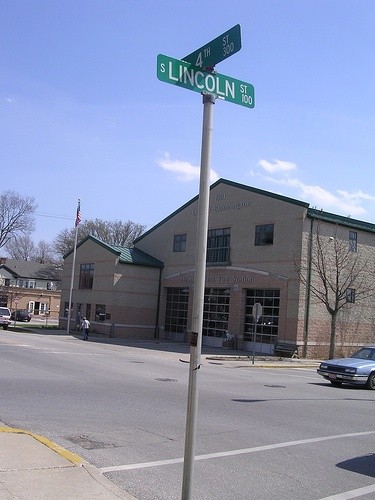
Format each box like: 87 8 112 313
0 307 12 330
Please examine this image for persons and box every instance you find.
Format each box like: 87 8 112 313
75 309 82 330
79 316 91 341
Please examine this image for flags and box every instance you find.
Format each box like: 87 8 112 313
74 202 82 227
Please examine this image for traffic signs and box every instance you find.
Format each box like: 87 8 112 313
181 24 241 69
157 54 255 109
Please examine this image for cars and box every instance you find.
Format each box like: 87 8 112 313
317 347 375 390
12 309 31 322
